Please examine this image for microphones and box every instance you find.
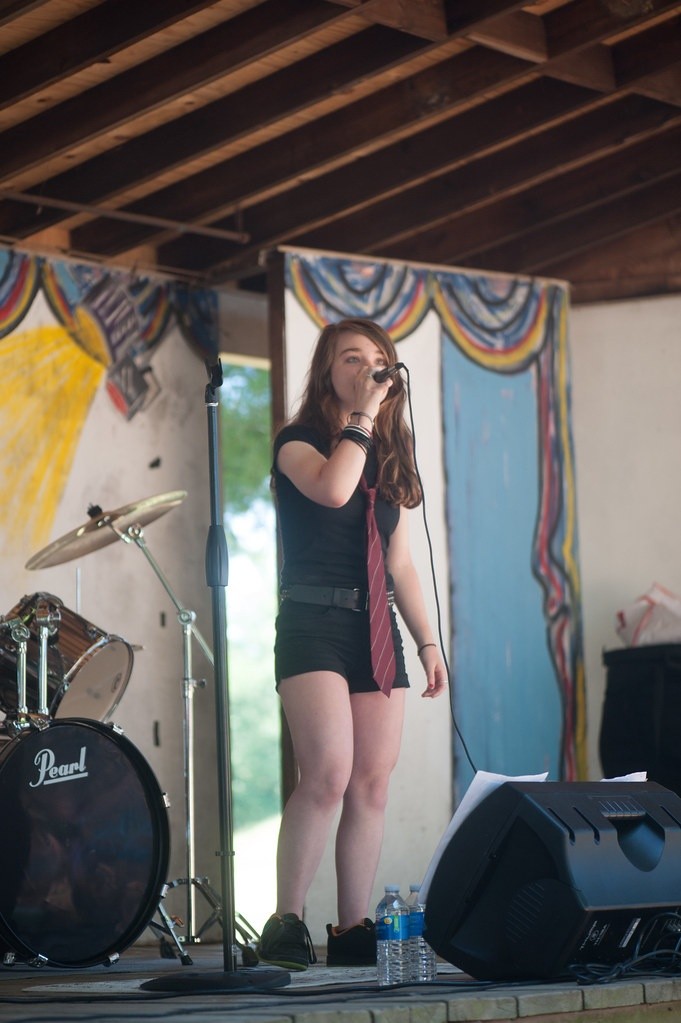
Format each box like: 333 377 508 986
374 363 404 384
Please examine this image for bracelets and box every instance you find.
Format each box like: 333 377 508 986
340 412 374 455
418 643 436 655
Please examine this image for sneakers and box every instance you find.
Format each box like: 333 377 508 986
256 914 316 970
327 918 378 966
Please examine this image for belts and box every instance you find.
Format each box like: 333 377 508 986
284 585 393 612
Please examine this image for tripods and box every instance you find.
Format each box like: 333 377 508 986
106 524 266 970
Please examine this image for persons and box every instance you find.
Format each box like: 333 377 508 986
252 318 449 969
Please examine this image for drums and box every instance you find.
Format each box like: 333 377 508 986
0 716 172 969
0 591 136 724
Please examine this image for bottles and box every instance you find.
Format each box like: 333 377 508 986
405 884 435 983
378 885 411 989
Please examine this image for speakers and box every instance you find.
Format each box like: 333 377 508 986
421 781 681 984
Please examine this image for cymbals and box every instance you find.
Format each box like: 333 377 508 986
24 488 190 571
